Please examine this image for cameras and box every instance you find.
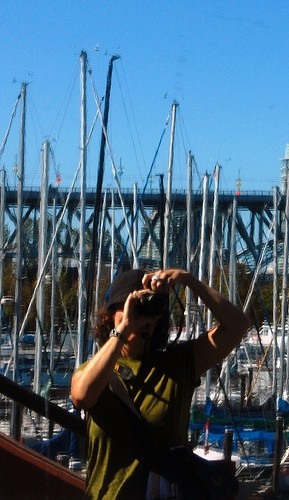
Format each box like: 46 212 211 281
139 290 169 312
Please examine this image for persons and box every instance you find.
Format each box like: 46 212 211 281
71 267 251 500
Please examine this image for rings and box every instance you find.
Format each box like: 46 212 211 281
151 276 159 280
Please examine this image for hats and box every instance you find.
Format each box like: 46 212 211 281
106 269 169 310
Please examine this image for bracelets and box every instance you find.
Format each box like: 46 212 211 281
109 329 128 344
